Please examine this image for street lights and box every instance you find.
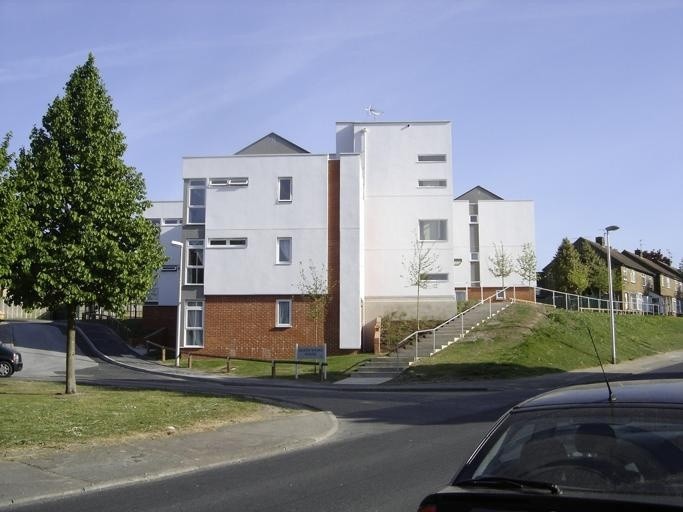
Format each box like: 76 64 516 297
170 239 185 369
603 224 622 366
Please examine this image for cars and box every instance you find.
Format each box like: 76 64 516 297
0 341 23 378
415 377 683 512
